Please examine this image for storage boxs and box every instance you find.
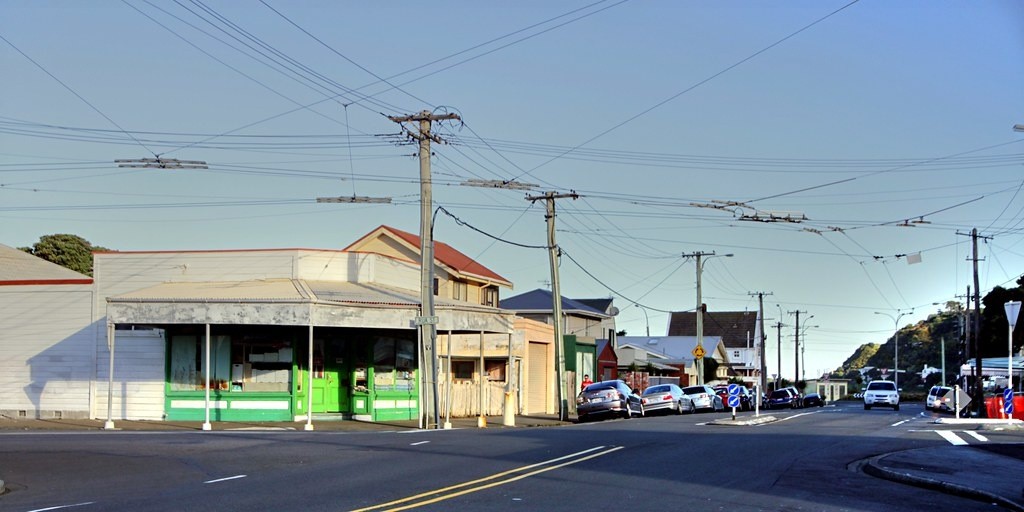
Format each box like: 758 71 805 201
249 348 292 362
246 364 292 383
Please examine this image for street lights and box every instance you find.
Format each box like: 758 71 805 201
875 311 914 388
802 315 814 380
696 253 734 386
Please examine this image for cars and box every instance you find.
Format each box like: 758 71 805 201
926 385 952 410
680 385 725 413
576 379 644 422
802 392 823 408
713 384 803 412
861 380 903 411
640 383 696 417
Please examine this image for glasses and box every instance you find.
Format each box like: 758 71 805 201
585 378 588 379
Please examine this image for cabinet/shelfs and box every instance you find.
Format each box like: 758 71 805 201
243 343 294 391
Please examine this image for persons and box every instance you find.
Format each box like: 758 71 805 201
740 392 754 412
799 390 803 408
581 373 594 391
